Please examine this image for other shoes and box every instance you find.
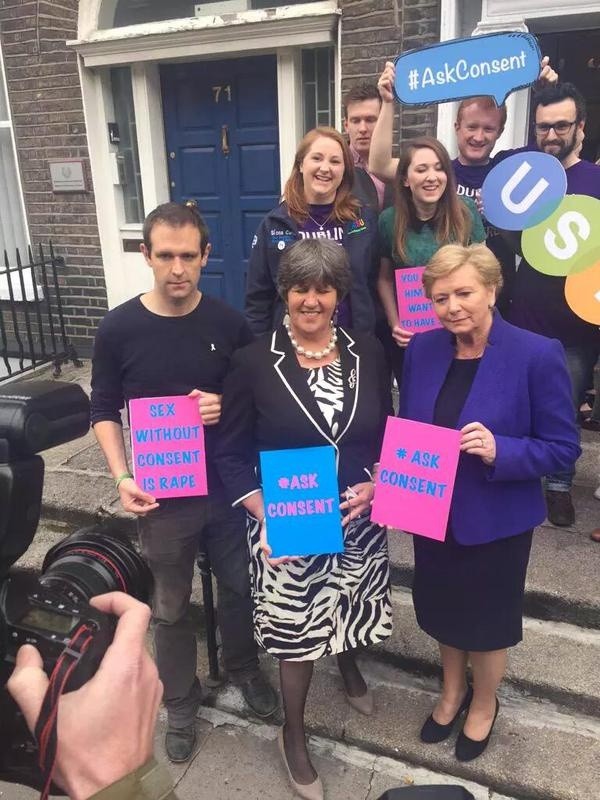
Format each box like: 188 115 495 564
545 490 575 527
592 486 600 500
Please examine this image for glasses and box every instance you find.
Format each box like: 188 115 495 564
532 119 579 136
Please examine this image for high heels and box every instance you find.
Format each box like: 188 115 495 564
338 665 373 716
420 679 473 744
455 698 500 762
277 721 324 800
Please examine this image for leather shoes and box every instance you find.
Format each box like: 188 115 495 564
219 652 280 719
165 723 196 763
590 528 600 542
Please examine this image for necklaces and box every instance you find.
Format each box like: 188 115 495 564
286 317 337 359
308 213 333 231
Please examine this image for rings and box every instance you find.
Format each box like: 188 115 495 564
480 439 487 448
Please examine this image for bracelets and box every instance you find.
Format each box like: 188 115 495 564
259 517 266 526
114 474 134 493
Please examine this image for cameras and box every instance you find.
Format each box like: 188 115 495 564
0 378 152 738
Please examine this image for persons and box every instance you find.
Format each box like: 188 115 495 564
89 202 280 762
242 126 378 341
217 236 396 800
370 246 582 762
342 56 600 541
7 591 181 800
